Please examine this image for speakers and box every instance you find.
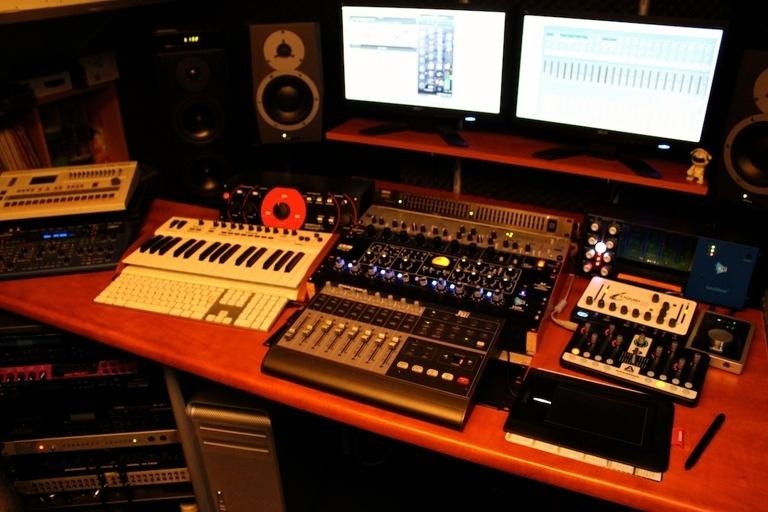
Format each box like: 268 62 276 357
250 20 326 145
710 47 768 247
138 24 247 208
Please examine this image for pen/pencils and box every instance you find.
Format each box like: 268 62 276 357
685 413 726 470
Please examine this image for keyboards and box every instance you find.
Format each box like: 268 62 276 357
93 272 290 333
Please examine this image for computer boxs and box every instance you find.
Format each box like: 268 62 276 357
185 389 309 512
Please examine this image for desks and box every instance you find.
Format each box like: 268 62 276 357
0 119 767 512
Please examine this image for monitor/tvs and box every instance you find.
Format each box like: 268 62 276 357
333 1 514 148
511 10 730 180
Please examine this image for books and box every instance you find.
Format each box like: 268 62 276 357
0 116 51 176
